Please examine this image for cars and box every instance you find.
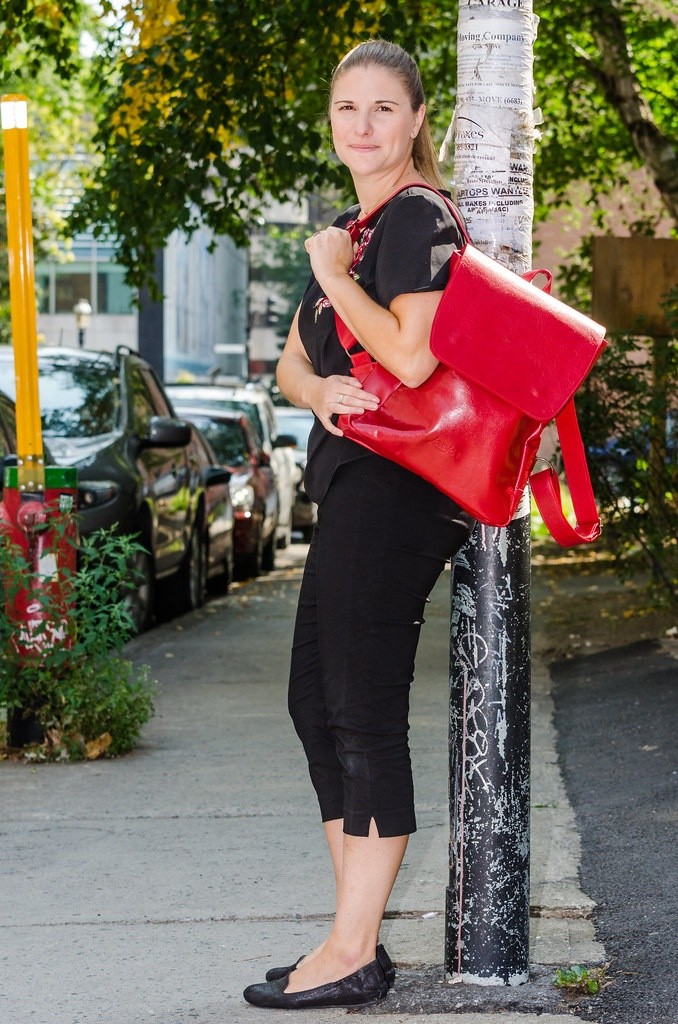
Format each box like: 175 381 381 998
0 343 316 647
586 410 678 498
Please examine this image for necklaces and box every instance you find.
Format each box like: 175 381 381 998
344 169 417 230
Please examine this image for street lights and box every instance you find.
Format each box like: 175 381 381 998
72 298 94 350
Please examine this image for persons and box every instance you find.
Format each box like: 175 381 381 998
76 299 90 349
243 40 477 1008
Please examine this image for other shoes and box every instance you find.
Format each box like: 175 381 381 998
243 959 388 1008
266 944 397 989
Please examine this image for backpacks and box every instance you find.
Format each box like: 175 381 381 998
334 181 609 548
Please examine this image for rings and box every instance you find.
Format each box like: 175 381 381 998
338 395 344 403
313 232 318 237
